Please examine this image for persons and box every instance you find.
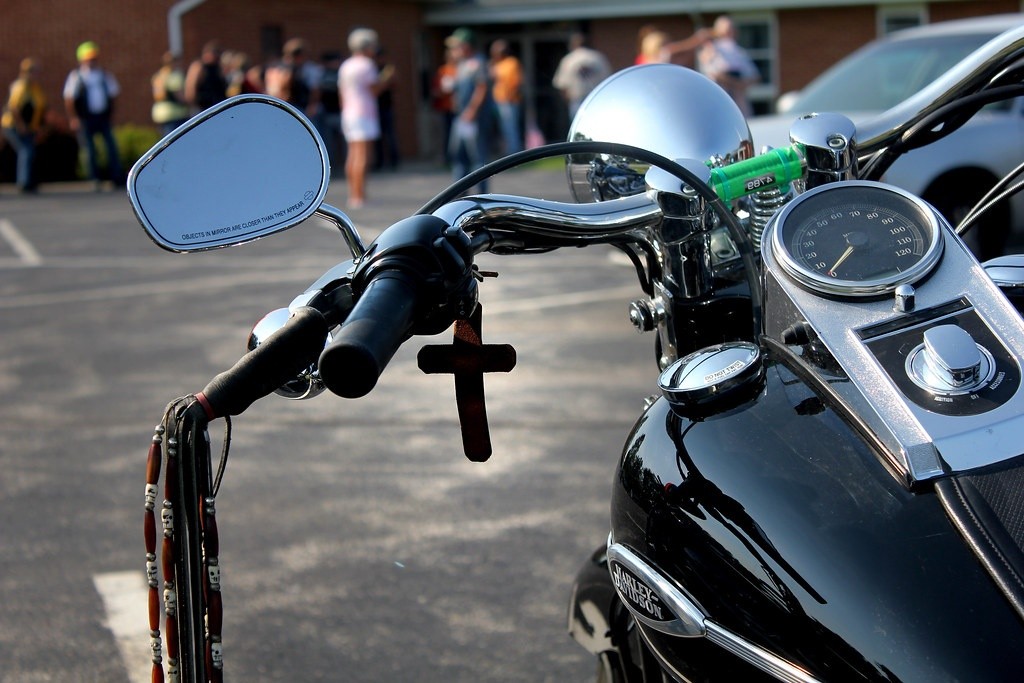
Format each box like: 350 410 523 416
65 41 127 190
2 57 45 195
141 23 760 195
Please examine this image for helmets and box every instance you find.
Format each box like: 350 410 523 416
77 40 100 61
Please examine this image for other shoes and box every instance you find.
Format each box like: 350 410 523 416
86 180 119 192
342 196 374 214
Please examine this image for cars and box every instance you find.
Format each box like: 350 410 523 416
746 17 1021 150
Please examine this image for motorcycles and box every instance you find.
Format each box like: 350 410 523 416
117 64 1024 682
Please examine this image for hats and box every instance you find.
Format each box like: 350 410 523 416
444 26 475 48
